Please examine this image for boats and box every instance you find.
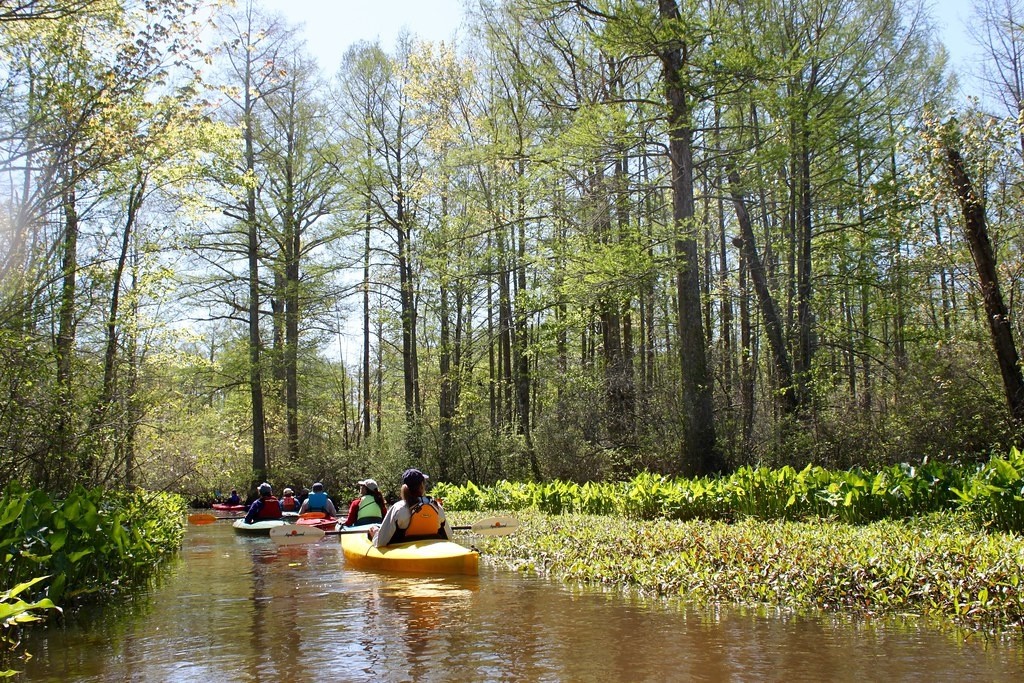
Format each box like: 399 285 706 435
212 502 246 512
282 511 338 531
338 523 480 579
231 517 291 536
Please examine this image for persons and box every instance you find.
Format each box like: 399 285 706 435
245 482 282 524
215 488 242 505
367 467 453 547
279 482 337 517
344 479 387 527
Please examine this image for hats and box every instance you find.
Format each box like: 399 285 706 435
402 469 429 483
283 488 294 495
358 479 378 491
257 483 272 492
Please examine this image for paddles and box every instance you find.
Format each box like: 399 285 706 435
268 515 520 546
188 511 327 525
312 498 443 528
